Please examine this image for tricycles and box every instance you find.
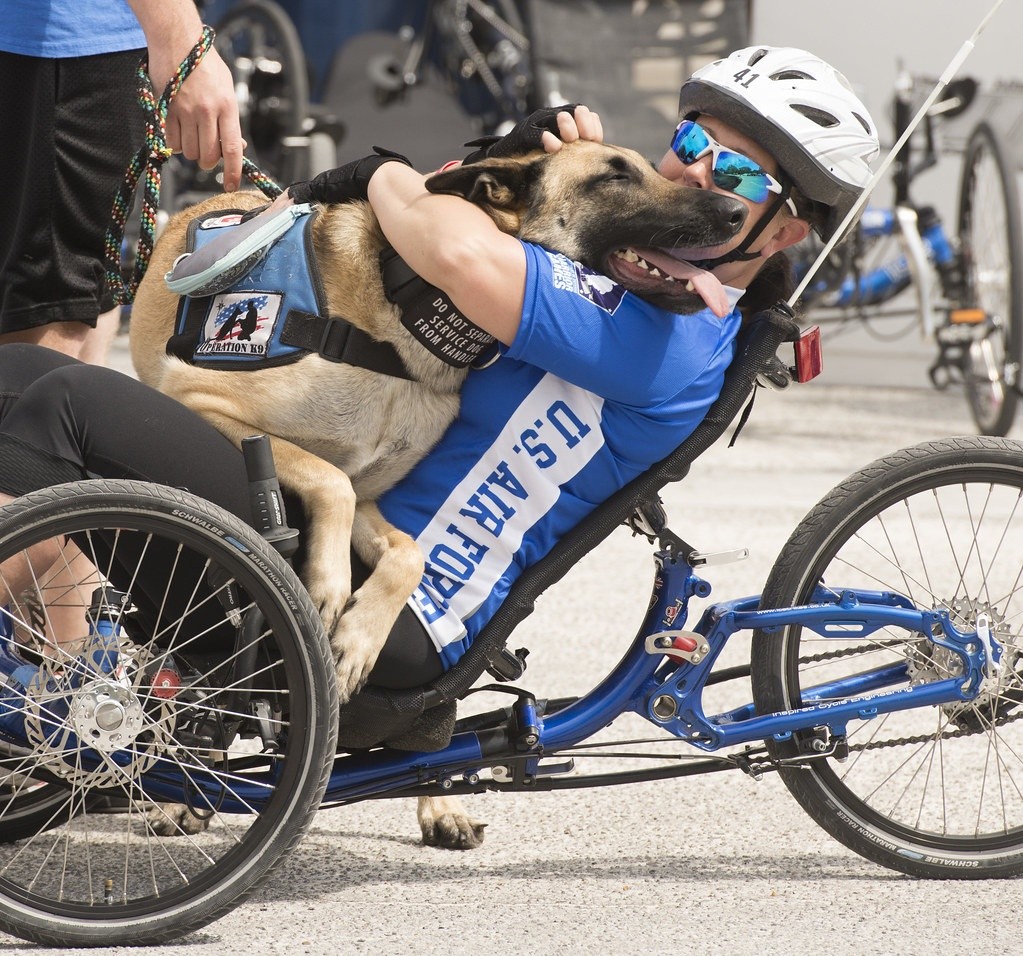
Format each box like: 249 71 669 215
0 291 1022 948
149 0 541 247
781 59 1023 439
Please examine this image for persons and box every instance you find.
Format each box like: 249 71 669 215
0 0 247 816
0 45 891 700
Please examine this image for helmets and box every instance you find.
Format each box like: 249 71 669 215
678 45 881 209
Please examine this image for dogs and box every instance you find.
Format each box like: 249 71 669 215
129 132 749 850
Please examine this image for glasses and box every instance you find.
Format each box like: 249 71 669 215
668 121 798 219
